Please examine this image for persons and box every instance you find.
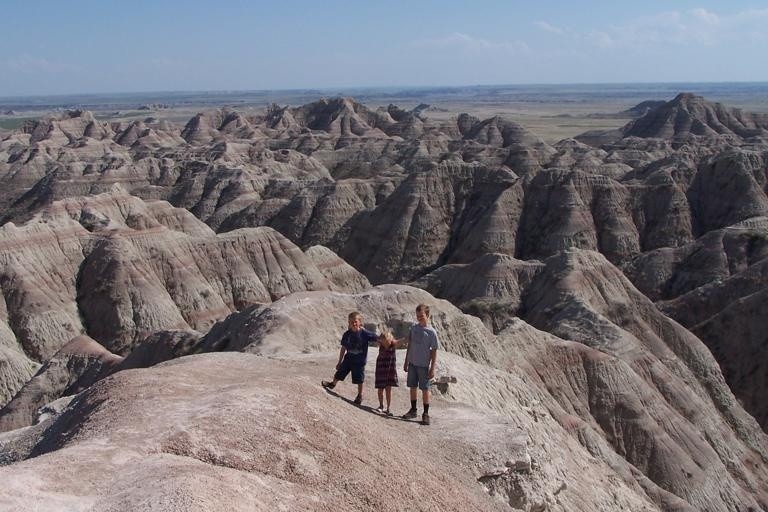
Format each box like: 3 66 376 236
374 332 402 415
321 312 380 403
404 304 438 426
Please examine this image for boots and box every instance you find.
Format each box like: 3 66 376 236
422 413 430 425
321 380 336 389
376 407 393 416
403 408 417 419
354 394 362 404
